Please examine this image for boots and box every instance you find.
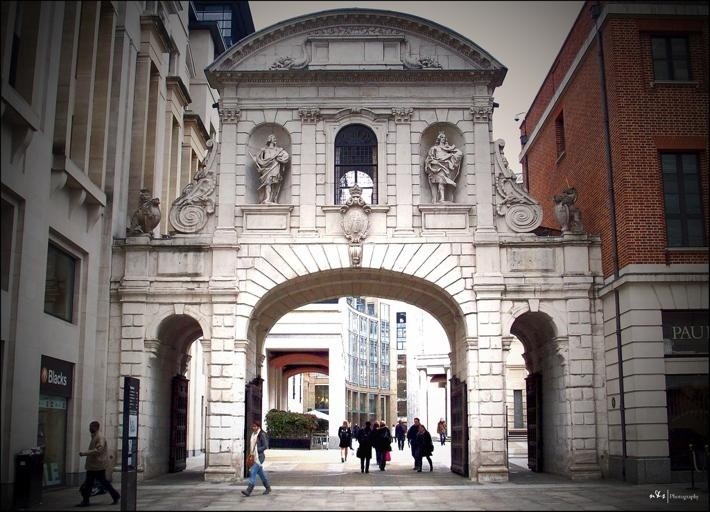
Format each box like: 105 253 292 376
241 485 252 496
263 482 271 494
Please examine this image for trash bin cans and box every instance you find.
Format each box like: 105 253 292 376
14 452 44 509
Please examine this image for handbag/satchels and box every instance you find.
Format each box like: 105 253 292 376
385 451 391 461
246 454 255 467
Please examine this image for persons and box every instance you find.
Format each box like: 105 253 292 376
252 134 288 204
240 420 271 495
423 131 465 204
436 417 448 446
74 420 121 507
338 417 433 472
